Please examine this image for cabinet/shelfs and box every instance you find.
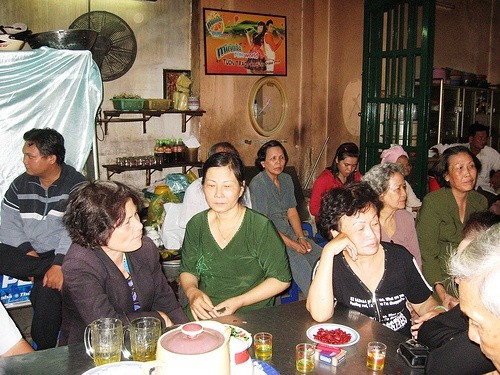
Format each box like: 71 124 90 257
413 79 499 148
101 110 208 186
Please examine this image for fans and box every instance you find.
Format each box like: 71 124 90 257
68 10 137 82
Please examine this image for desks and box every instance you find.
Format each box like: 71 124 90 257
0 298 425 375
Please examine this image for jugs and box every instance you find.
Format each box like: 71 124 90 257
143 223 162 249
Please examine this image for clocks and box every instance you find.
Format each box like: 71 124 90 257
248 76 288 138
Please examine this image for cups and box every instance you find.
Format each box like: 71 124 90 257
366 342 387 371
295 343 315 373
254 332 272 361
115 155 156 167
188 96 199 110
122 317 161 361
83 318 124 367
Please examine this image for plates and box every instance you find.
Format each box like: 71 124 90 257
306 323 361 348
162 260 181 267
252 359 281 375
225 324 252 354
80 361 153 375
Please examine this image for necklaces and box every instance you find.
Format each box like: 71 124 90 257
216 216 238 243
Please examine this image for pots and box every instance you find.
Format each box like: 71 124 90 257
154 324 231 375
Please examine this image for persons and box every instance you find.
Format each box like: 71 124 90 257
0 301 35 358
0 127 87 351
178 124 500 375
179 152 292 323
61 179 190 346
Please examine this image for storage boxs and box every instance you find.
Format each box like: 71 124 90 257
311 346 347 366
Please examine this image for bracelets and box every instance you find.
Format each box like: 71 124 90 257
434 306 448 312
297 237 307 240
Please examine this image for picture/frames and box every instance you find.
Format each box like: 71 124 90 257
203 7 288 77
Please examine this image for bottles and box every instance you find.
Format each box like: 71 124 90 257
154 138 185 166
